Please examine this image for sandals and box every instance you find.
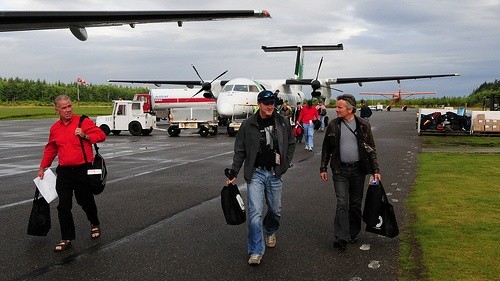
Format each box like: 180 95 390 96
55 240 71 252
91 226 100 238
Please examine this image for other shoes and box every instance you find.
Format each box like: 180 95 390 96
309 147 312 150
305 145 309 149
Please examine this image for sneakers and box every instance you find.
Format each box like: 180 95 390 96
249 254 262 264
265 232 276 247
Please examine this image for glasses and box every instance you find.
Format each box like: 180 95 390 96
259 95 275 100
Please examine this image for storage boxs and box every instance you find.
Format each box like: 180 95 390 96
472 114 500 132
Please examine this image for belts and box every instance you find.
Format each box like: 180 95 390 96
257 166 274 171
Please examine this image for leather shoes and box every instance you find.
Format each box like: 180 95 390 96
334 240 347 250
349 234 358 243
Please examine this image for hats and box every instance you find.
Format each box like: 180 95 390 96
360 100 365 103
258 91 276 104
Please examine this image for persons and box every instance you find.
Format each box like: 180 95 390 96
273 90 327 144
37 95 106 252
359 100 369 120
224 91 295 264
320 94 381 251
295 101 320 151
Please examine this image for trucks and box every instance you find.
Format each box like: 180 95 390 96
95 88 221 138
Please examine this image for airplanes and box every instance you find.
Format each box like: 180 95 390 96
106 43 460 138
358 84 438 112
0 6 273 41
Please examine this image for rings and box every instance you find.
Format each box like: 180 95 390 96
77 131 79 133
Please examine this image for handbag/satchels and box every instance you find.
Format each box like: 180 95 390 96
221 168 246 225
88 154 107 194
362 149 374 174
364 180 383 224
27 187 51 236
365 200 399 237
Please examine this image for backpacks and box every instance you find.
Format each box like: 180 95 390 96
364 107 372 116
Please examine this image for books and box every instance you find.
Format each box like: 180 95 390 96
33 168 58 203
